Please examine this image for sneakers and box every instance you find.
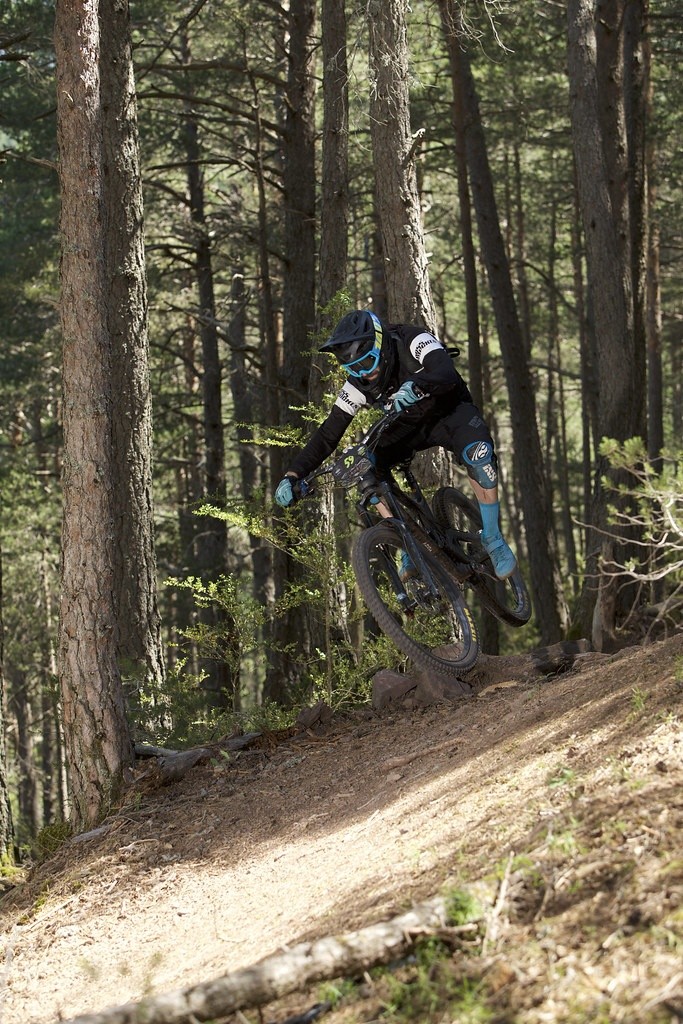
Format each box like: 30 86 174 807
481 529 518 578
398 552 418 582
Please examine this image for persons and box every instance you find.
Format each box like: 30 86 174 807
275 310 519 581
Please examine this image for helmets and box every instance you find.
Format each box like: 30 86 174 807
317 309 394 396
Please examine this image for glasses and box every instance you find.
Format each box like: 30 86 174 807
340 351 380 377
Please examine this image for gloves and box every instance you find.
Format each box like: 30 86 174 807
385 381 425 411
275 475 300 508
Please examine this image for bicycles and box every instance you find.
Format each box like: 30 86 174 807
280 388 533 675
